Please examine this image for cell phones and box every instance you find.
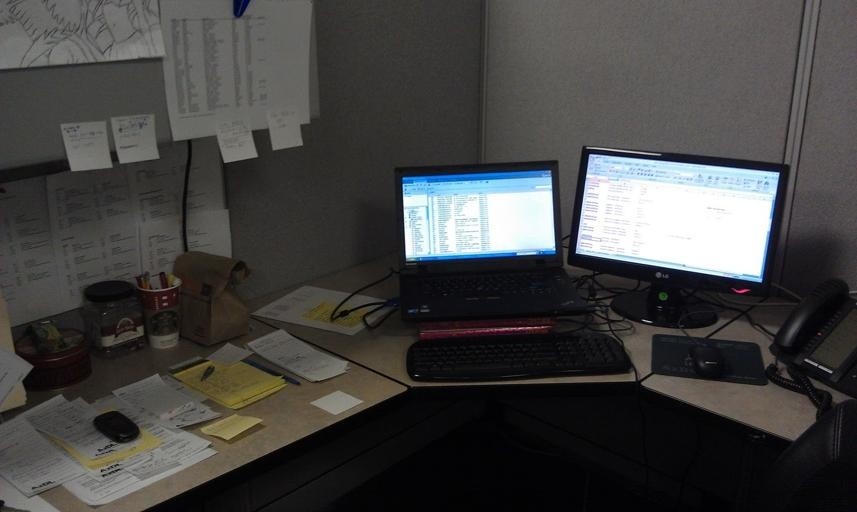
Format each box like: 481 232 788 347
93 411 140 444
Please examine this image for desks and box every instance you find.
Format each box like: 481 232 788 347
0 254 855 511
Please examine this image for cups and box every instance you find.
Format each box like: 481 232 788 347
137 274 183 350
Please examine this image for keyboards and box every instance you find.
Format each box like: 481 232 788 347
406 334 631 379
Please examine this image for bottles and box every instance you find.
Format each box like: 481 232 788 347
81 280 147 360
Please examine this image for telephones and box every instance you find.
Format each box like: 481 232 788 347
768 279 857 398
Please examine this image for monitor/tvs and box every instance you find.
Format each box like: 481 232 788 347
566 147 790 326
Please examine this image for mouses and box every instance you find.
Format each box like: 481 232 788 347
691 345 724 378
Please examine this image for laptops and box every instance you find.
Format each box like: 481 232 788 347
395 161 586 322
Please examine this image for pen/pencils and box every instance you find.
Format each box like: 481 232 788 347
136 271 174 290
244 358 301 386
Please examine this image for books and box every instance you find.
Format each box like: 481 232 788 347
250 284 395 336
